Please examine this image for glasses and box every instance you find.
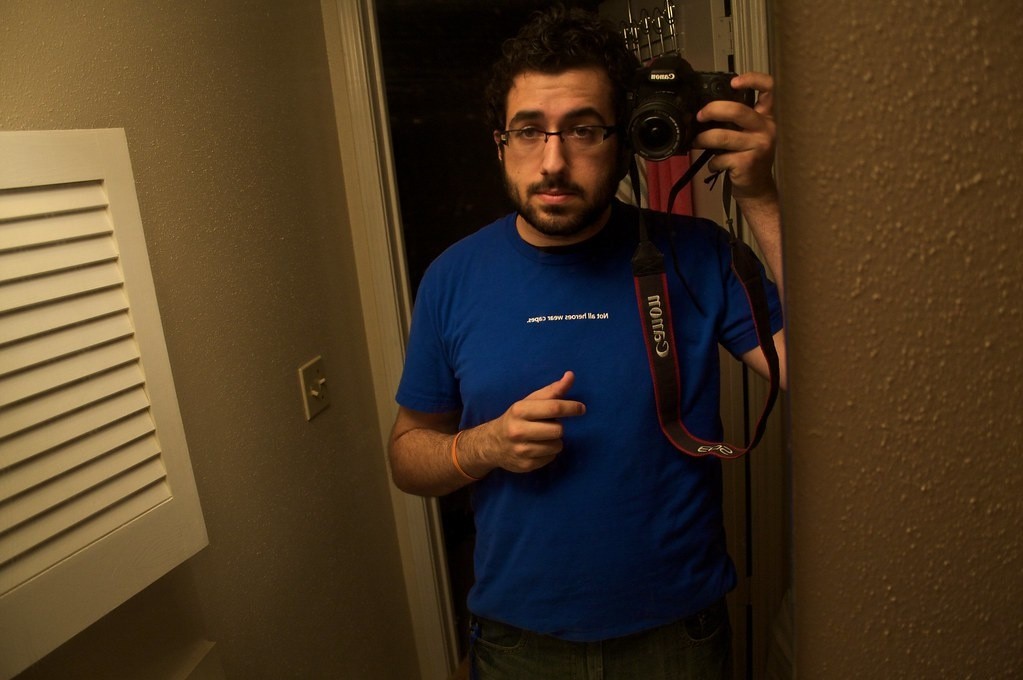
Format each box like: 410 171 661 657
499 124 621 150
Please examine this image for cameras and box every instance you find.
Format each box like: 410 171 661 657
614 47 758 163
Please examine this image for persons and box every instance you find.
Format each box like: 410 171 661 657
385 8 787 680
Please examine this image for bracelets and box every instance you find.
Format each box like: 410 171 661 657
451 430 481 481
